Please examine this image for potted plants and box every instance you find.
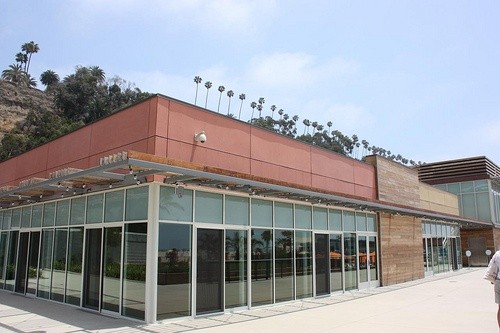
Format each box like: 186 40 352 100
158 265 185 285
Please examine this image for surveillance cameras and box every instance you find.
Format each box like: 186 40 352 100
199 133 207 143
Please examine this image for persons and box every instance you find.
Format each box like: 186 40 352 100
486 250 500 333
